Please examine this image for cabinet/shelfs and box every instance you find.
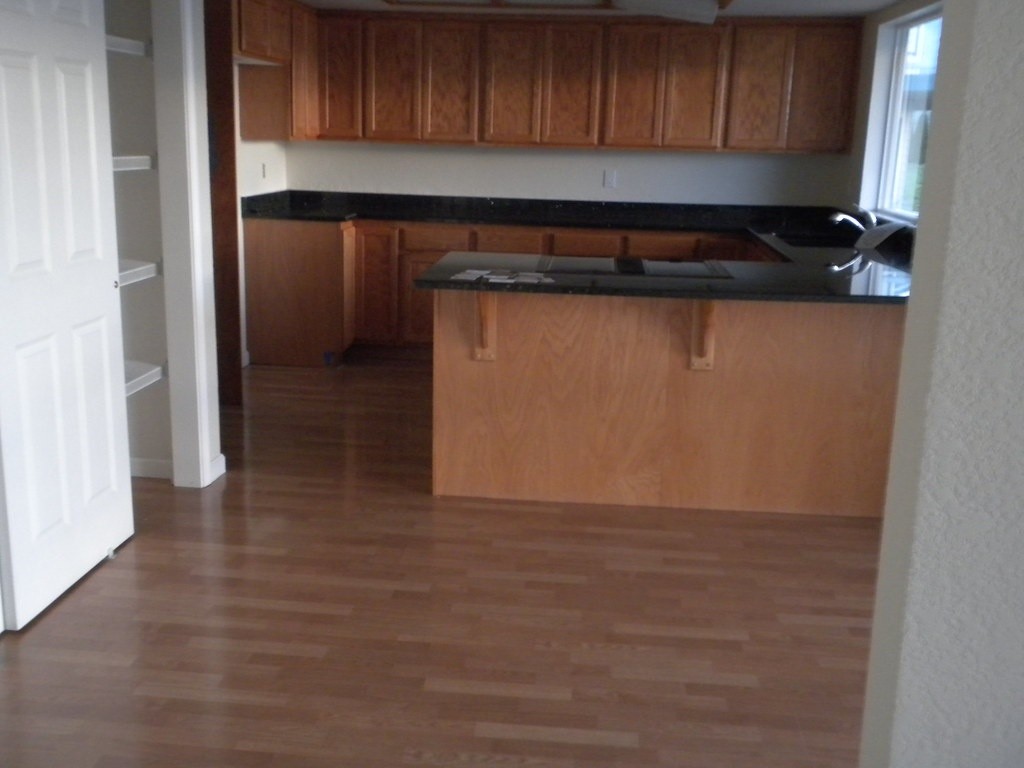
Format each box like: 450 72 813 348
356 226 755 351
0 0 227 635
232 0 861 156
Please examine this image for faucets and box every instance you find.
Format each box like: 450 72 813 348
830 201 877 233
827 252 874 279
